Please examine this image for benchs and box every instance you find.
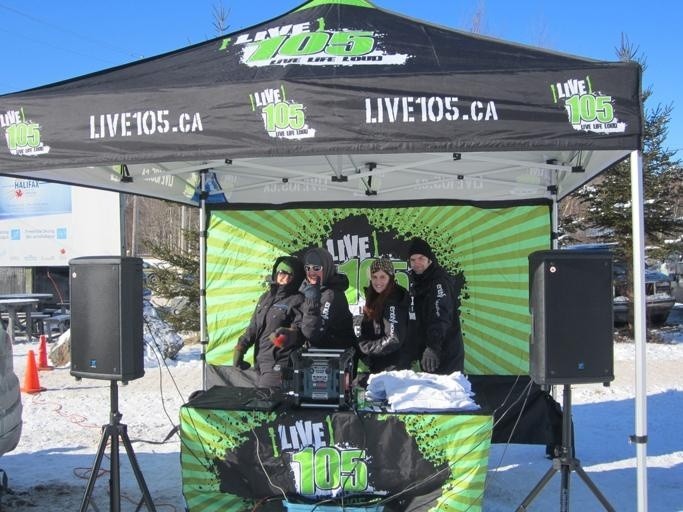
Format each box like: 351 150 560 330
0 293 71 344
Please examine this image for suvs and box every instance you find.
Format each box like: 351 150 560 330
561 244 674 326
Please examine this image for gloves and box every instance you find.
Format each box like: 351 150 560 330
421 348 440 373
269 327 296 347
232 345 250 369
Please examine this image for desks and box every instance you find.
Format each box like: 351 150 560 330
179 386 495 512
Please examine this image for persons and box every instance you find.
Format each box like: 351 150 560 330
407 238 465 375
232 256 304 392
298 248 358 350
356 258 412 373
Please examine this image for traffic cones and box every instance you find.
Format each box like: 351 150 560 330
36 335 52 369
19 350 46 394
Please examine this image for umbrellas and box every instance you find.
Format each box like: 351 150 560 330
646 271 668 296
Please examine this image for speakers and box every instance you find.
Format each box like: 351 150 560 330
68 256 145 385
528 249 615 391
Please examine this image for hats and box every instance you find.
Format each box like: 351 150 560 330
272 238 431 289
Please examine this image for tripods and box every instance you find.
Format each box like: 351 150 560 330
79 380 156 512
514 384 616 512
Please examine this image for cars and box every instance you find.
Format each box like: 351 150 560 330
666 260 682 307
0 322 23 492
